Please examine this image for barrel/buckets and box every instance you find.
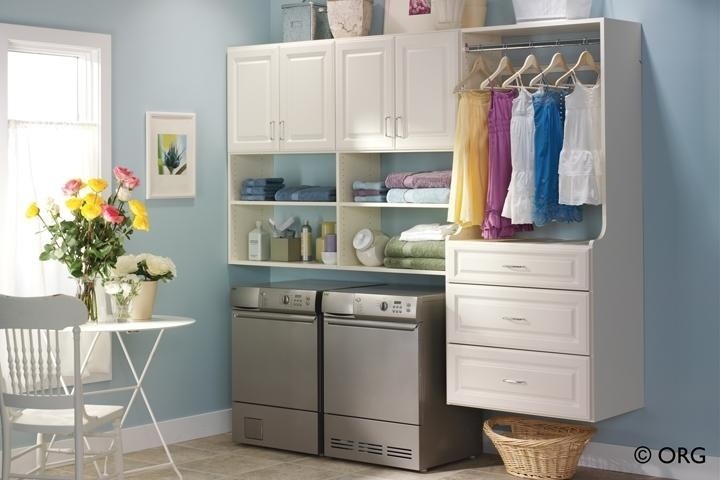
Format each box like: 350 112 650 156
110 280 158 320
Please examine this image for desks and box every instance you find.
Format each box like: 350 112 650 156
51 315 196 480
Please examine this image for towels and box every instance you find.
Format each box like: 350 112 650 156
385 219 454 268
349 170 449 204
241 174 334 201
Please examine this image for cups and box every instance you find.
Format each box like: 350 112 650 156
315 222 337 264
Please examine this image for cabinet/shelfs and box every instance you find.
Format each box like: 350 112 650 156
336 27 455 276
442 18 645 424
228 38 337 268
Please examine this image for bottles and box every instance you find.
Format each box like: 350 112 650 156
247 220 272 261
353 229 392 266
301 219 313 261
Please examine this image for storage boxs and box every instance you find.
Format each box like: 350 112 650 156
281 1 333 43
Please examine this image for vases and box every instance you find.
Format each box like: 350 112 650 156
111 297 130 323
75 278 95 321
128 280 153 319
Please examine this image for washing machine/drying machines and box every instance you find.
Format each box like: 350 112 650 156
321 284 482 471
230 279 385 455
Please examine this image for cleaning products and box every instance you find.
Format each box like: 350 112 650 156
248 221 270 260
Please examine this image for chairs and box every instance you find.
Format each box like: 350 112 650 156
0 294 122 480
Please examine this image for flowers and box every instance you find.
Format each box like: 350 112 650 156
112 254 176 283
24 164 150 280
103 277 140 309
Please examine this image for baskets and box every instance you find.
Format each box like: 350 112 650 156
484 416 597 479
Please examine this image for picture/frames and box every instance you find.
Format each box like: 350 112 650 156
144 112 199 198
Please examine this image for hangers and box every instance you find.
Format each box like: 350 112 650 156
449 40 600 92
